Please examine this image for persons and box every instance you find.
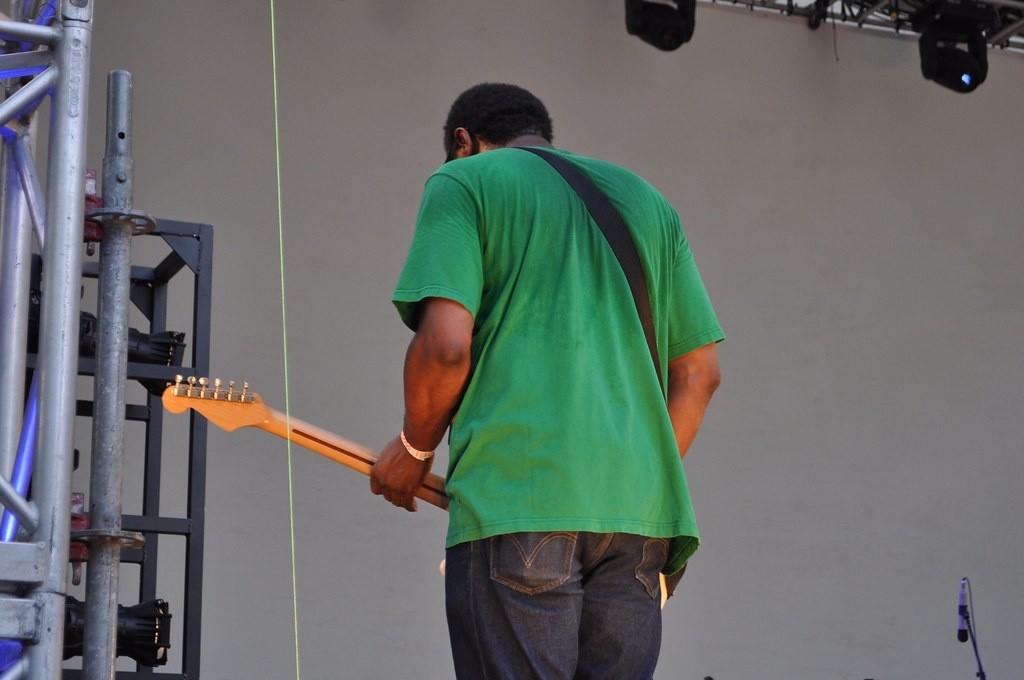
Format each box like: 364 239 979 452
372 83 725 680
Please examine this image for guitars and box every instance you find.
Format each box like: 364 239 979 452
161 374 686 611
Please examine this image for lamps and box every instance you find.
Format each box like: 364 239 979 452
624 0 696 52
918 28 988 94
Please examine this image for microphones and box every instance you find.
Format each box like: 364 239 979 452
958 578 969 642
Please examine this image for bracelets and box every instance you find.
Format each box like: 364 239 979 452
400 431 435 461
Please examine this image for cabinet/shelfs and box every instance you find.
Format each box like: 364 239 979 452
20 216 213 680
